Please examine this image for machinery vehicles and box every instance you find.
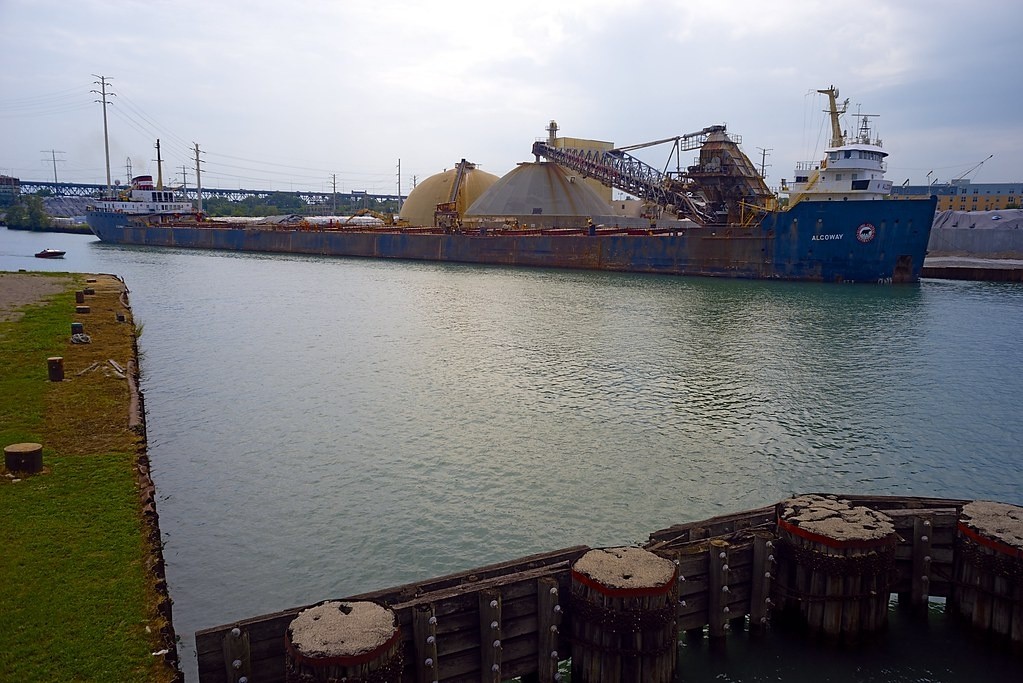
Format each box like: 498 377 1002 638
344 208 394 226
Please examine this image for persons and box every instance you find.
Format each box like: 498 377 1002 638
502 218 521 230
586 217 593 226
441 218 463 235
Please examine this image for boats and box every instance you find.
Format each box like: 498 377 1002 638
34 248 66 258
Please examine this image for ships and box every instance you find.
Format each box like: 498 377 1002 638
85 85 939 284
